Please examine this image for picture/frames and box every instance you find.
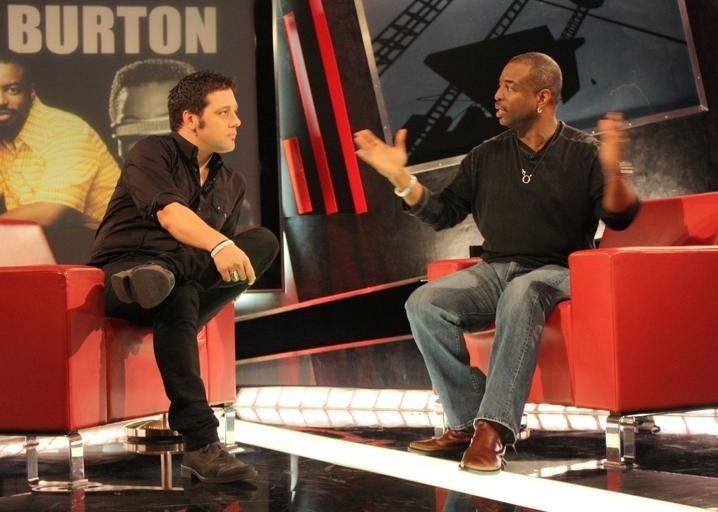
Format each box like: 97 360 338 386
351 0 712 182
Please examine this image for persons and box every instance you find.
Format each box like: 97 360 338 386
1 50 123 267
106 57 205 163
88 71 282 487
353 52 641 474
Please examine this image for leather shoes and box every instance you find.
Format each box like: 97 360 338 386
183 480 258 512
406 426 476 457
458 418 510 471
177 441 260 486
108 261 178 312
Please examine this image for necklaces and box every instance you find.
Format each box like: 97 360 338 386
511 124 562 184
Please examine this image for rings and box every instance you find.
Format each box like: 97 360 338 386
230 270 239 283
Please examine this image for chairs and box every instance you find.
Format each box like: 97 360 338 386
1 218 250 494
424 192 718 471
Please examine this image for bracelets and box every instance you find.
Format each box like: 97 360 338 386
390 177 416 198
209 239 234 257
